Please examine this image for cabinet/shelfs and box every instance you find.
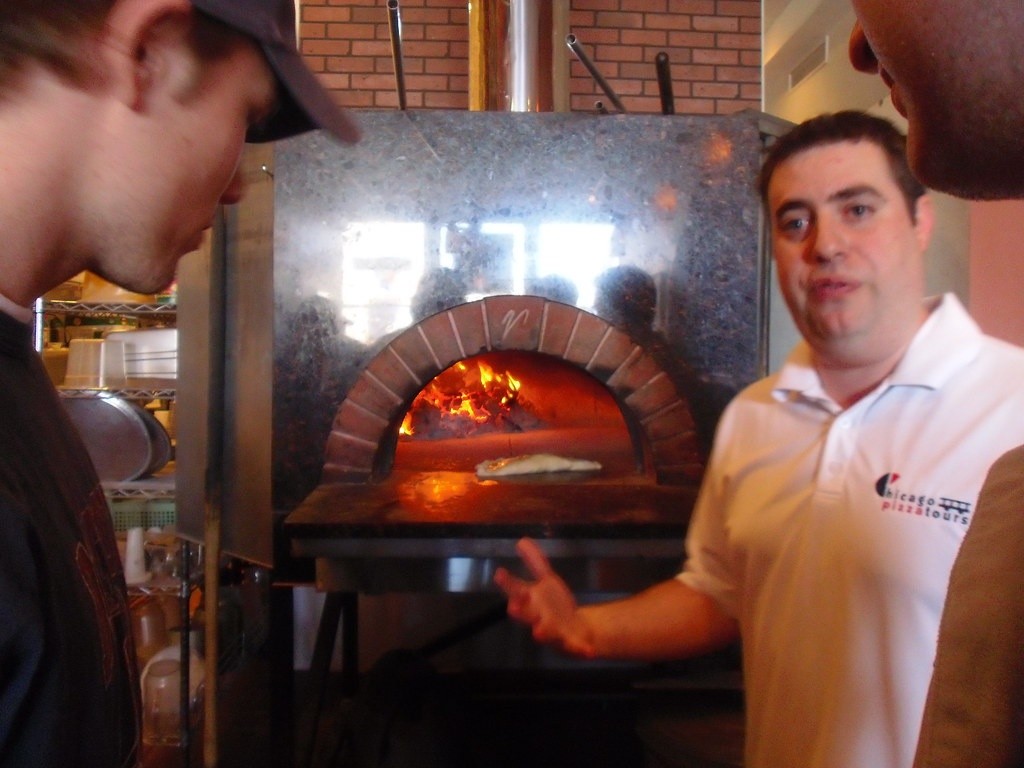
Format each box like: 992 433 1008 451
33 301 246 768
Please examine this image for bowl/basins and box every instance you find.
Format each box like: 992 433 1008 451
65 338 127 390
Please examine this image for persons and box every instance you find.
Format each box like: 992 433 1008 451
493 109 1024 768
849 0 1024 768
0 0 358 768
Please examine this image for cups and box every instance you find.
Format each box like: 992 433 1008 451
168 625 204 658
144 659 188 747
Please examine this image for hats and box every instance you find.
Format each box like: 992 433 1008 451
192 0 360 147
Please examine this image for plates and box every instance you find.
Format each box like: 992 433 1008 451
57 391 151 484
126 401 172 476
